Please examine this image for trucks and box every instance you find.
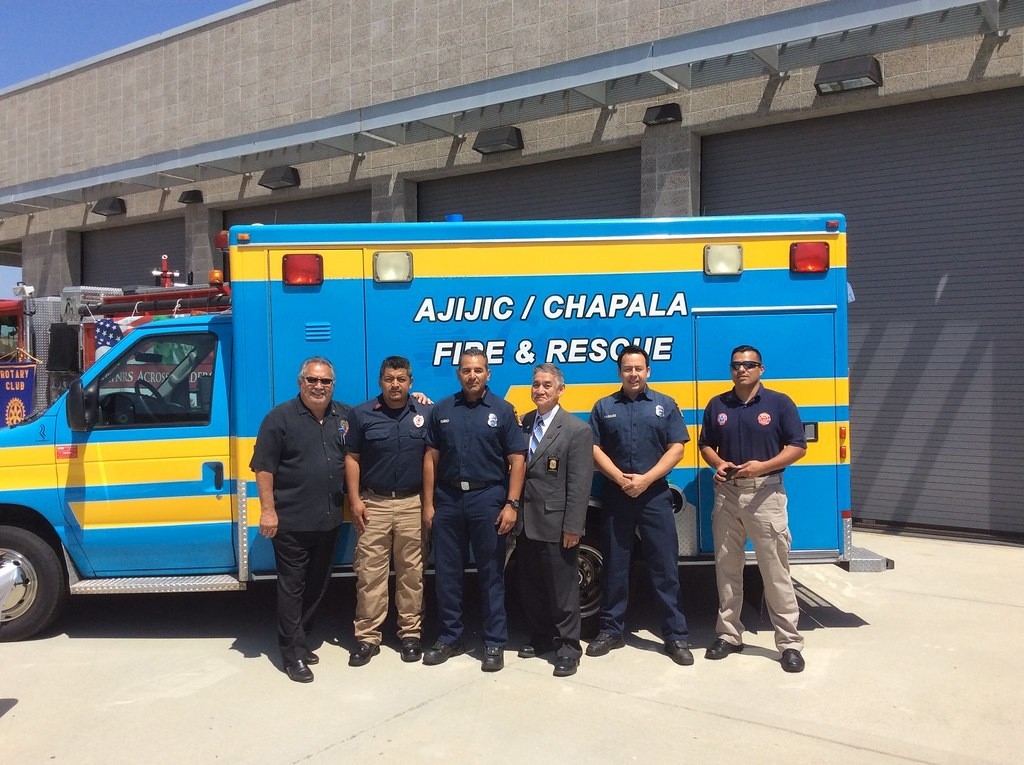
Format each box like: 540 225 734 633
0 204 896 642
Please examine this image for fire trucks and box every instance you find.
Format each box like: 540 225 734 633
0 255 236 398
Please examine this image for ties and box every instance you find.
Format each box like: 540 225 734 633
529 416 545 463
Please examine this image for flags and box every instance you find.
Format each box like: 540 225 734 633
94 316 153 364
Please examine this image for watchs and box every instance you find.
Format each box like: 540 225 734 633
505 500 520 509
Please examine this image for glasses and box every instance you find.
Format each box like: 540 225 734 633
302 377 333 384
730 361 760 368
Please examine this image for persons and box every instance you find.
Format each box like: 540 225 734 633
697 345 807 672
345 356 435 666
586 345 694 665
518 363 594 676
249 357 434 683
423 348 529 671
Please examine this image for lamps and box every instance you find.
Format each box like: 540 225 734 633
472 126 524 155
258 165 300 189
91 197 126 216
814 54 883 96
178 190 203 204
642 103 682 126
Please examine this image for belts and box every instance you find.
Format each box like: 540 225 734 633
726 478 778 487
441 481 494 490
367 488 420 498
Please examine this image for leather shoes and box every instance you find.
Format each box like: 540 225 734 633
586 633 625 657
482 646 504 672
423 641 465 665
400 638 421 661
519 641 553 657
305 653 319 664
706 639 744 659
781 649 805 672
286 659 314 683
553 657 581 677
349 643 380 667
664 641 694 666
